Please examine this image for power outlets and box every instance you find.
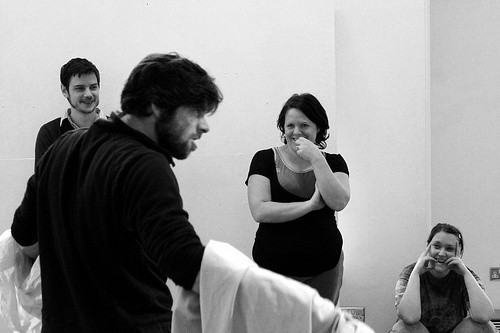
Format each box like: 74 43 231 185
490 267 500 281
342 306 365 323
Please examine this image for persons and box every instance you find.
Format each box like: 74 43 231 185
34 56 112 171
10 50 225 333
244 93 351 309
387 221 499 333
